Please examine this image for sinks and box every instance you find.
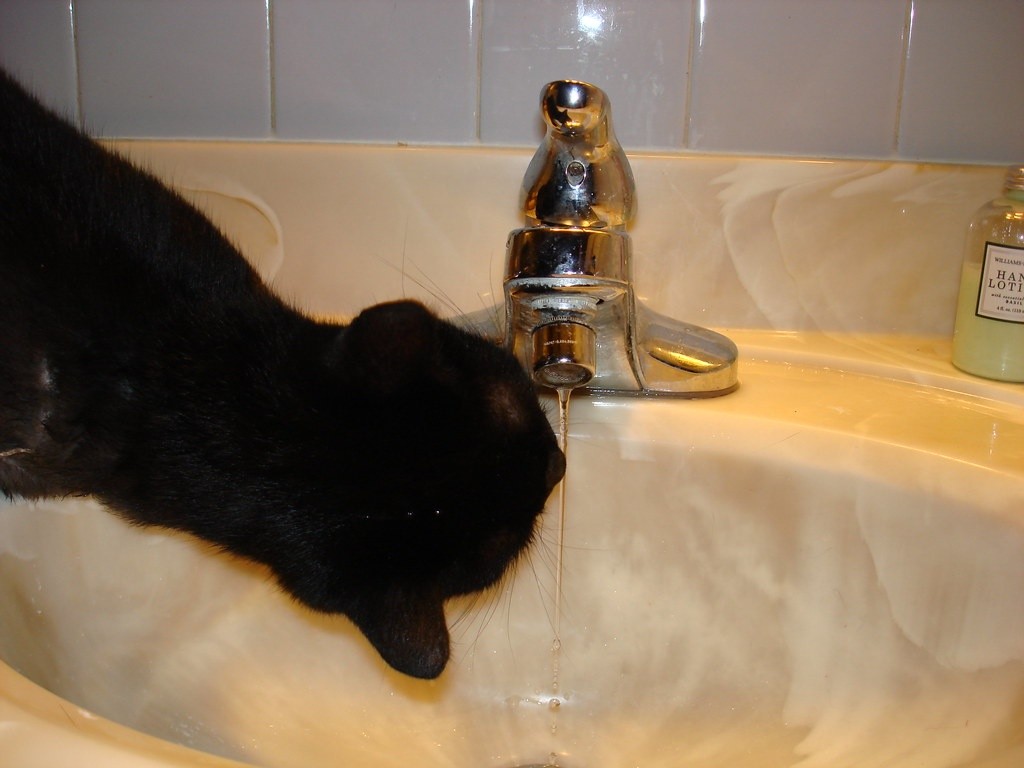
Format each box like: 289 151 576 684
0 372 1023 768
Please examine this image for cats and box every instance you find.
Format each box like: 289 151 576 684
0 67 567 680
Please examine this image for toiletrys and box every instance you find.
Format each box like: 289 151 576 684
949 161 1024 382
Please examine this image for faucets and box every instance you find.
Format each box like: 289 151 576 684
498 78 645 396
